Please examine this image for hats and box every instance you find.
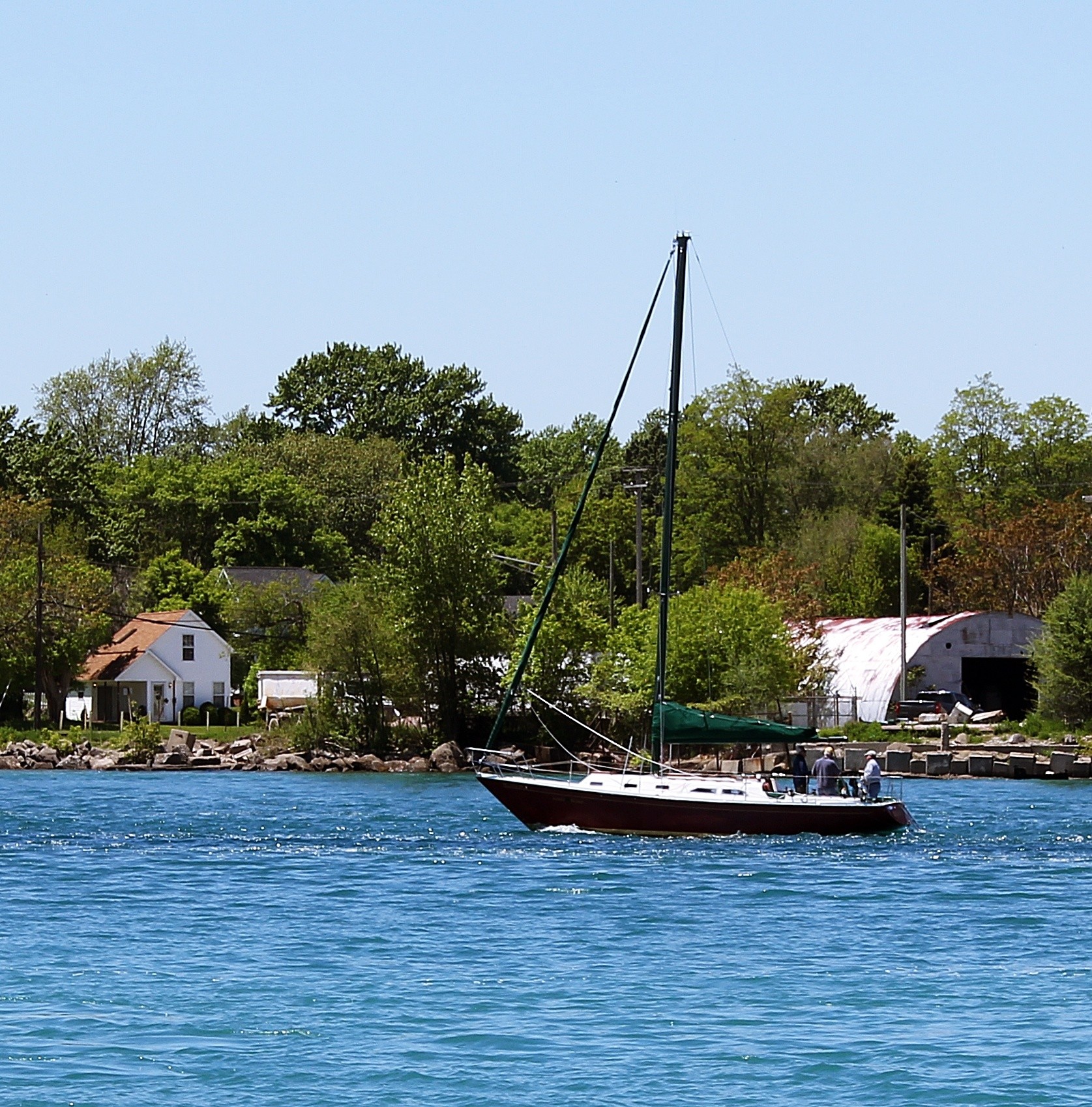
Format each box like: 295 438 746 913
864 750 876 756
824 747 833 755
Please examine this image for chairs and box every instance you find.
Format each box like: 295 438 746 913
777 778 819 794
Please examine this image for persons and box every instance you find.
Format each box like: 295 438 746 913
794 745 810 794
811 746 843 796
860 750 881 798
768 754 800 794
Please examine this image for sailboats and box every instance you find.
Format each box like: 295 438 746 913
477 228 913 835
